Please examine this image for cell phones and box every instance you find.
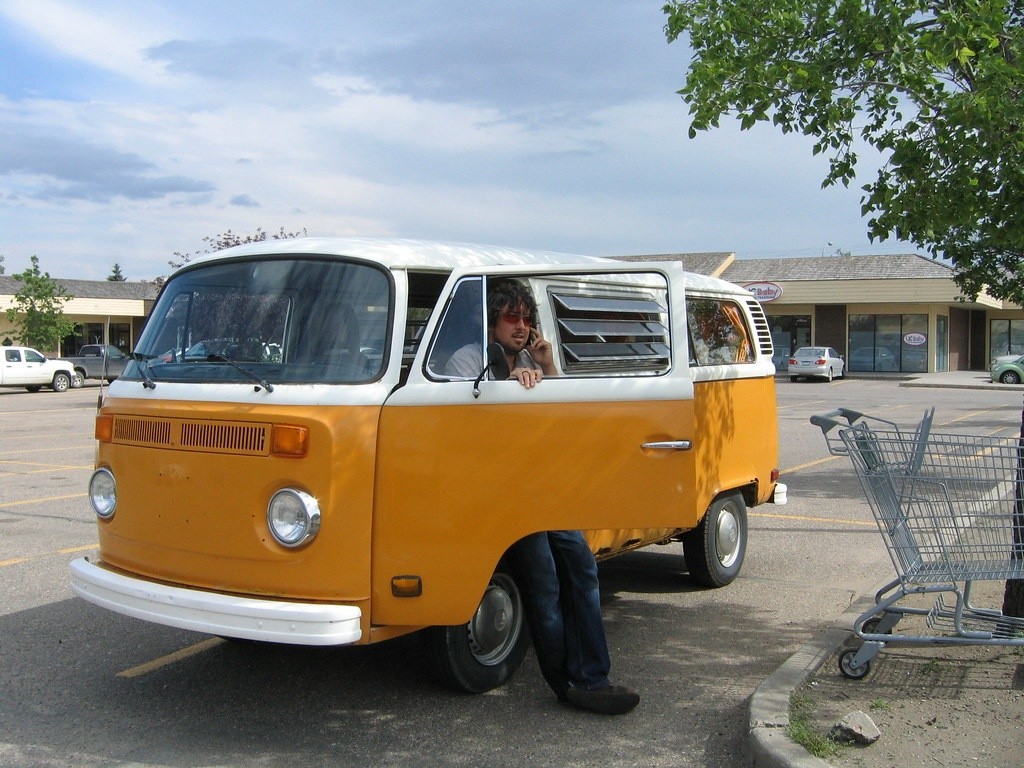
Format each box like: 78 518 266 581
526 332 533 346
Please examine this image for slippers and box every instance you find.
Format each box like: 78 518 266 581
561 680 640 714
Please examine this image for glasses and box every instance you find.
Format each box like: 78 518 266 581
500 311 533 325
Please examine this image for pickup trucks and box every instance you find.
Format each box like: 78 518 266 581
43 343 131 389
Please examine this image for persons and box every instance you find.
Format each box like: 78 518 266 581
444 276 640 715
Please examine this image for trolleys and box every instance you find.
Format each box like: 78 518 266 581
810 407 1024 682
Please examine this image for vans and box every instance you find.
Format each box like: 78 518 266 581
67 237 788 695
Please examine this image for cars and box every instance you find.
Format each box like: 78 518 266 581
848 346 896 372
149 333 283 365
989 355 1024 385
788 347 846 382
0 345 77 392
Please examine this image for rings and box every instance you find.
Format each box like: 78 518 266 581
522 370 529 375
